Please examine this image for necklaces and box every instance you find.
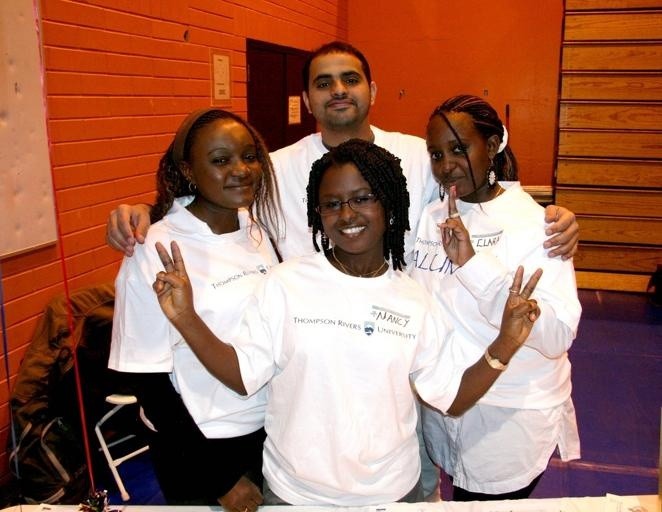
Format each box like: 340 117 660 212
493 186 503 199
330 243 387 279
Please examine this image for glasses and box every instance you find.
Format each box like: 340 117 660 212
315 192 383 217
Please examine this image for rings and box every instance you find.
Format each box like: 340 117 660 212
507 287 521 295
449 212 460 219
243 507 248 512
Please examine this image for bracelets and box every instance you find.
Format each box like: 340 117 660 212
483 344 511 374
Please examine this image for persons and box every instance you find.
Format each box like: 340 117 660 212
150 135 543 512
402 92 584 512
104 39 581 512
107 109 282 512
